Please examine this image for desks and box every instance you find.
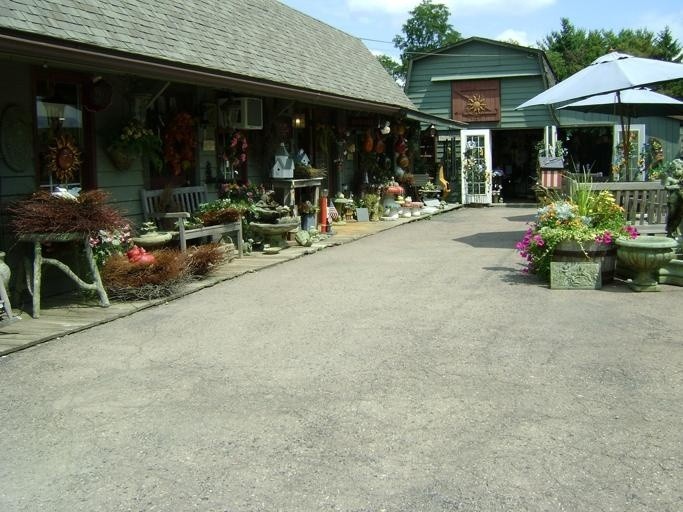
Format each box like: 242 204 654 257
270 177 324 214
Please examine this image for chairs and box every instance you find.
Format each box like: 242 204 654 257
138 184 244 262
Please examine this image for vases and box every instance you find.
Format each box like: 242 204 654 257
548 236 604 290
492 192 501 202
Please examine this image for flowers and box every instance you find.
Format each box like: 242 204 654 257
517 161 636 271
490 169 503 195
224 133 247 166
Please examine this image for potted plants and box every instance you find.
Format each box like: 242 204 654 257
401 196 412 217
200 198 241 225
413 202 423 217
365 192 380 222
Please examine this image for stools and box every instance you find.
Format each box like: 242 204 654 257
21 232 111 318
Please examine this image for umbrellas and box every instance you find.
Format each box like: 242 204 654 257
555 88 683 182
512 52 683 182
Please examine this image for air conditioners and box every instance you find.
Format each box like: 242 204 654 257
220 96 263 131
